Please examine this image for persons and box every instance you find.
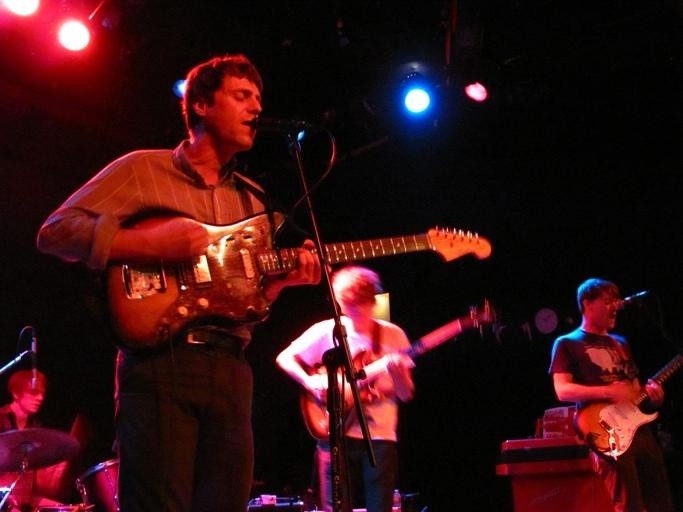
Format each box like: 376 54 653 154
0 369 47 433
275 264 416 512
548 277 674 512
35 53 332 512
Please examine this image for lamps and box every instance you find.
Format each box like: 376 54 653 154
385 58 443 124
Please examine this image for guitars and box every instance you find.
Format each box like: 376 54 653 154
301 304 496 442
101 207 492 348
573 355 683 459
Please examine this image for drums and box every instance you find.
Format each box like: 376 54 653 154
38 505 94 512
75 458 120 512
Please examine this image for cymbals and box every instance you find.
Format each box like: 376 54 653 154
1 429 78 472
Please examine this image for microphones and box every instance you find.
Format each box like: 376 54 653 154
250 113 306 132
31 329 38 390
609 290 647 311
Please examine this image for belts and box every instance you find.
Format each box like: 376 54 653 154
177 328 245 358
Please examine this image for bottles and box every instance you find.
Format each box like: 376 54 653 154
392 489 401 511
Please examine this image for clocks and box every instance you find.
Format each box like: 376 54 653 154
533 306 559 335
